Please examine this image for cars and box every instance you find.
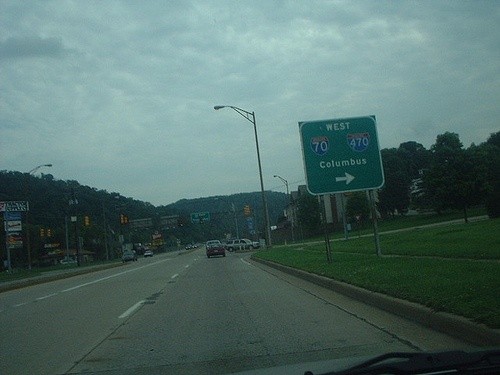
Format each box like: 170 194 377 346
144 250 153 257
205 239 260 258
120 250 138 263
185 244 195 250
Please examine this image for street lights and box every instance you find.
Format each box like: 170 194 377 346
273 175 296 244
213 105 272 249
68 187 81 266
24 163 53 271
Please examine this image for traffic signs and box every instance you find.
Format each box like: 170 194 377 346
298 114 385 197
